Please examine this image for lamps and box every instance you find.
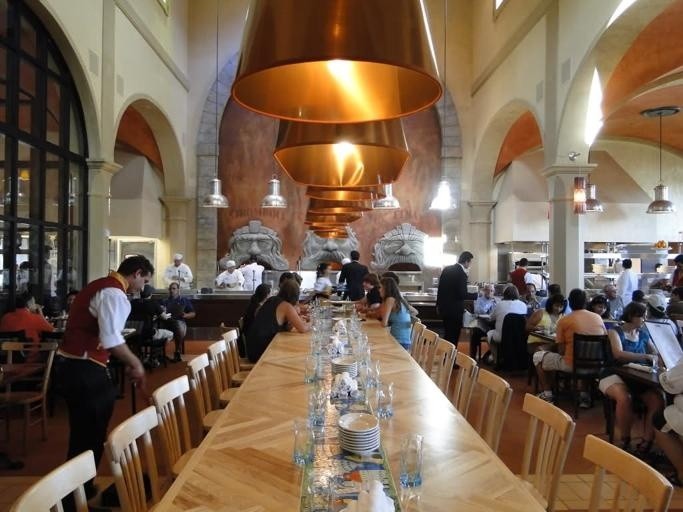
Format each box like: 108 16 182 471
584 146 602 213
639 104 680 214
201 1 458 213
571 174 586 215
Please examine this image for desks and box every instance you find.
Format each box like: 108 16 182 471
156 306 542 508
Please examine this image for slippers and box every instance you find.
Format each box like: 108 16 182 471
667 472 683 487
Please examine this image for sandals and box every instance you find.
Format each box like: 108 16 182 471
636 437 652 458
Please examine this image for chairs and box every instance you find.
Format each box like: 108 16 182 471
521 393 576 508
104 405 171 508
208 340 242 403
239 316 252 360
434 338 457 396
582 434 674 510
220 329 253 385
10 449 98 510
407 321 427 360
452 351 478 418
418 329 439 377
469 313 570 396
476 369 512 452
189 353 226 430
405 315 422 347
547 311 681 441
151 373 201 479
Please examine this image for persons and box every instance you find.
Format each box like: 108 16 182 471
436 252 482 369
56 255 153 504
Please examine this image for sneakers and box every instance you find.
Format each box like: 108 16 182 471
536 393 555 405
1 454 24 470
151 359 160 366
578 397 591 408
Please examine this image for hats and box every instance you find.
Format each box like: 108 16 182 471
174 254 182 259
141 285 154 295
226 260 235 267
341 258 351 265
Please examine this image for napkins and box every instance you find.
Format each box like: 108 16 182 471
327 372 359 399
327 319 347 357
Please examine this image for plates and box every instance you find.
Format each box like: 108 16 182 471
591 263 607 274
330 318 349 346
338 413 381 456
331 354 362 380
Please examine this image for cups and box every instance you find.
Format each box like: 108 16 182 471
399 432 425 488
354 318 395 417
292 301 326 471
298 475 335 512
